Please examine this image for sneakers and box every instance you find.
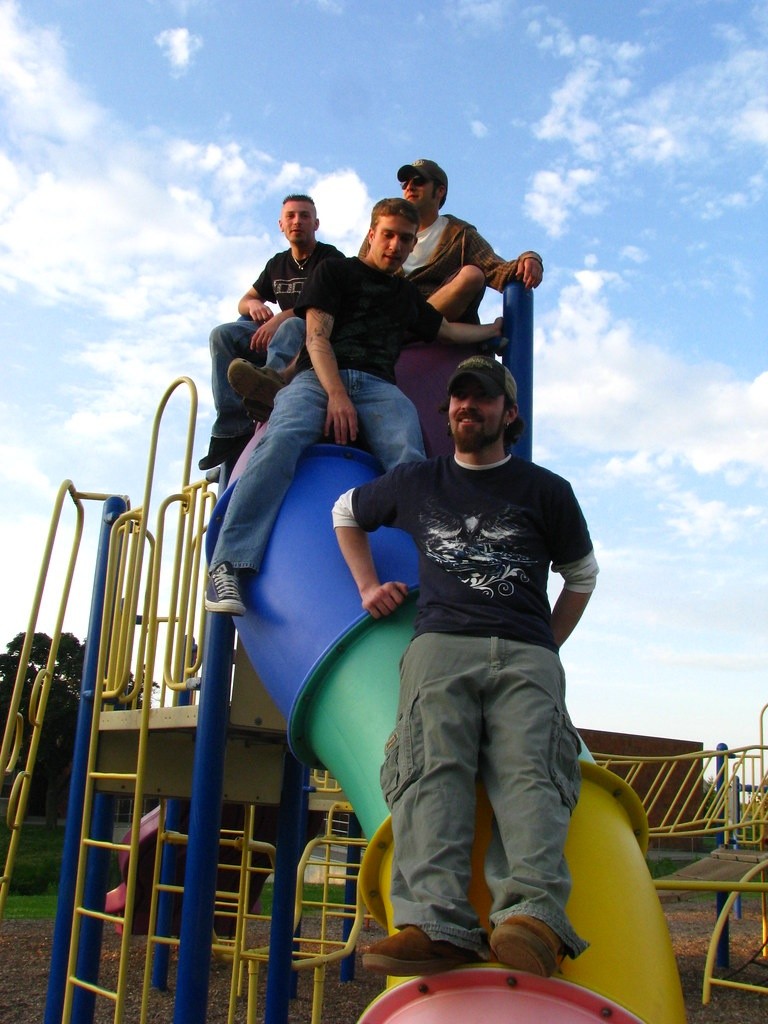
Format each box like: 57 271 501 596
206 560 246 615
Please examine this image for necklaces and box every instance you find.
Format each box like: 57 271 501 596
291 240 317 270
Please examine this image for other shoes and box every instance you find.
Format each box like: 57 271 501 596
362 924 472 974
227 359 283 407
242 398 269 419
199 423 254 470
489 915 569 978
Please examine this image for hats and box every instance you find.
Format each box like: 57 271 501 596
397 159 448 190
447 355 518 405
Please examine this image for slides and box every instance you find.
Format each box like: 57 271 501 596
102 802 278 938
202 340 689 1024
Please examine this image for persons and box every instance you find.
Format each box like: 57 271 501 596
205 197 503 617
331 355 600 979
198 159 545 470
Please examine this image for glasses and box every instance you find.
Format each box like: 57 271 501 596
400 176 437 188
